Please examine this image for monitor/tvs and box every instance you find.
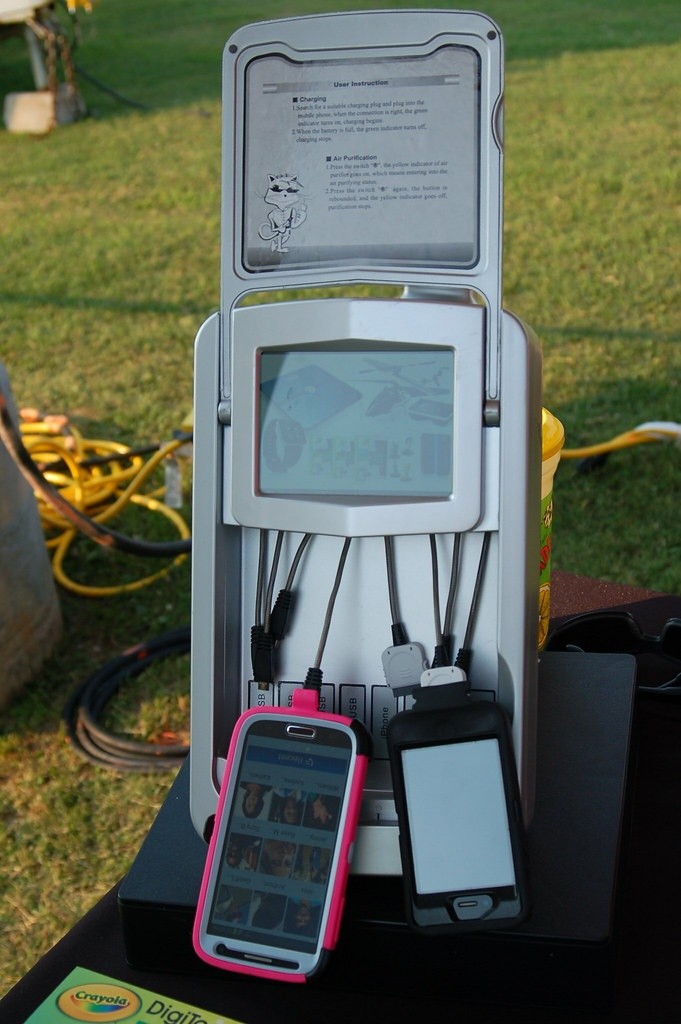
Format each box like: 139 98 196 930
224 298 483 539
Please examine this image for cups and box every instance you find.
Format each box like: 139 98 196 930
539 410 565 651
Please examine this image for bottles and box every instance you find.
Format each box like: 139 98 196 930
163 452 183 511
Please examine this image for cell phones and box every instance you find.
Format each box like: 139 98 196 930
193 704 369 983
385 700 530 932
